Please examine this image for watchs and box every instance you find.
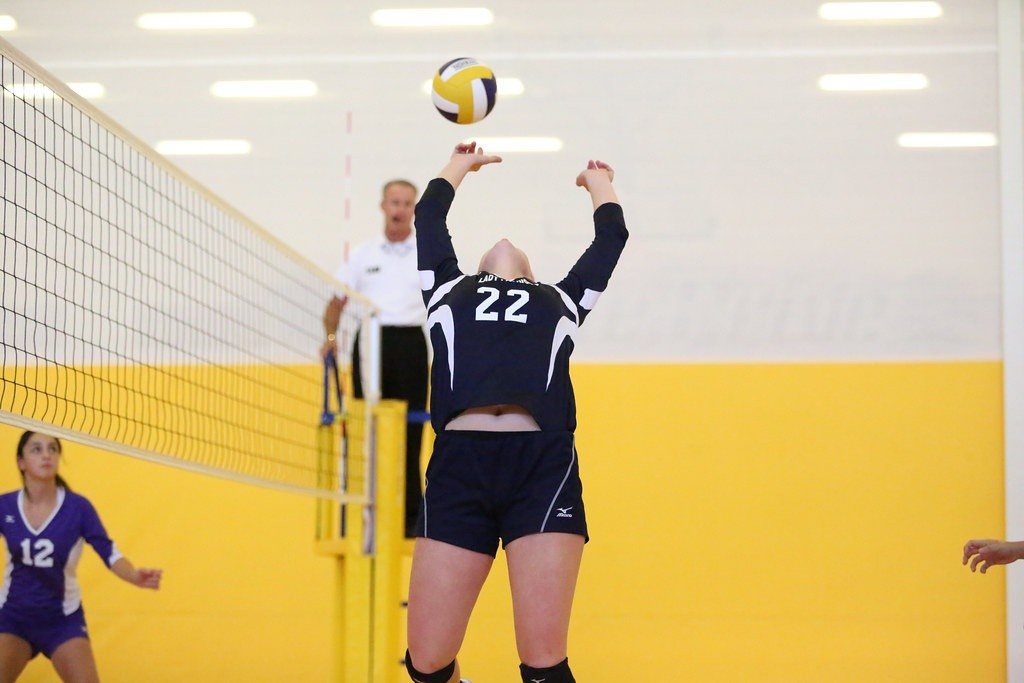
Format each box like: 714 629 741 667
327 333 336 340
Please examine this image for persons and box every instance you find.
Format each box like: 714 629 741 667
402 142 629 683
319 180 429 541
962 539 1024 574
0 431 162 683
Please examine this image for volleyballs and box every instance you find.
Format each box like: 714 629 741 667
430 54 499 125
